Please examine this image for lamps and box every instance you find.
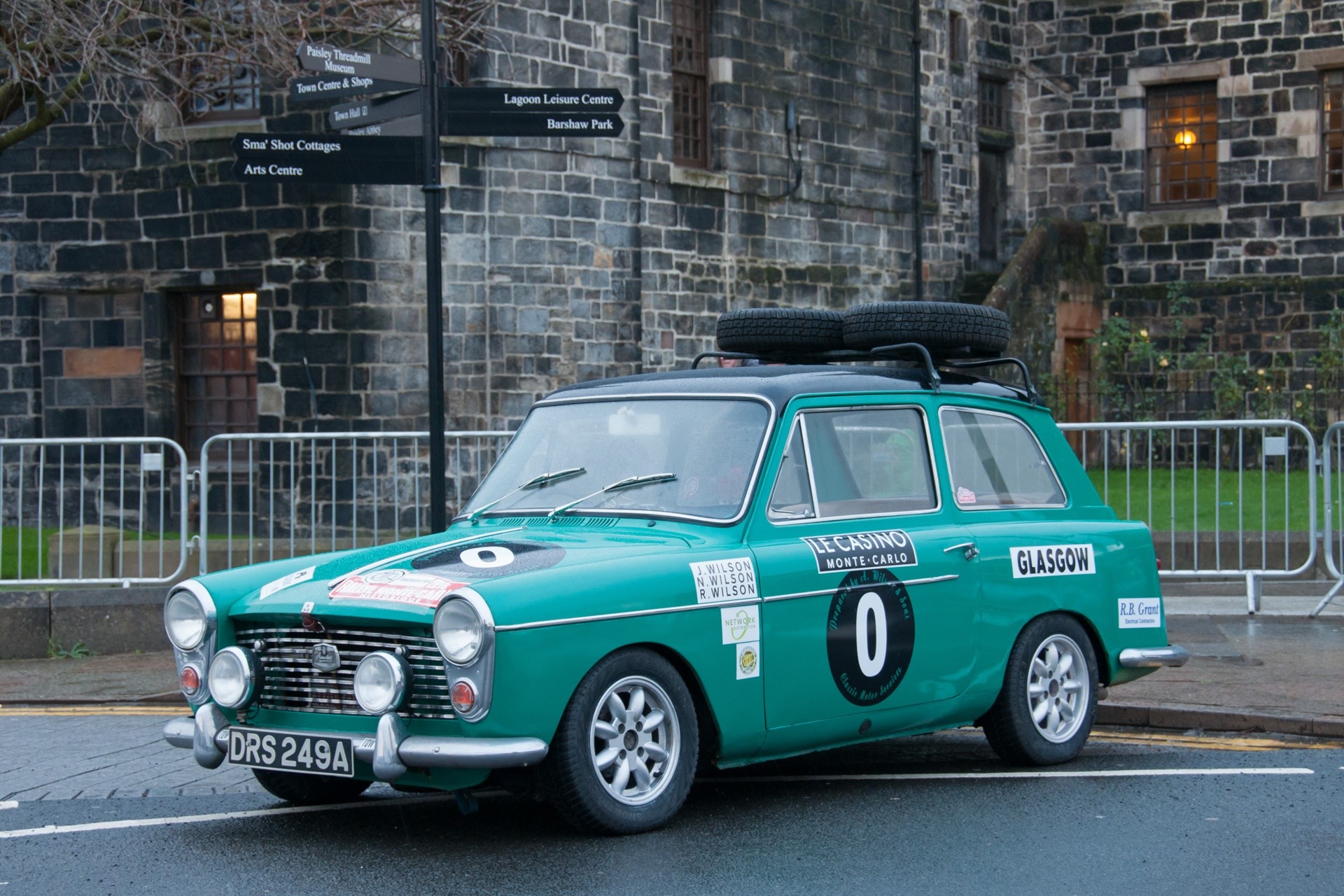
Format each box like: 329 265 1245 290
1174 121 1195 150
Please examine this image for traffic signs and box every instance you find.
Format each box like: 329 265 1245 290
435 86 629 139
230 133 427 188
296 42 426 87
290 75 420 101
323 90 423 131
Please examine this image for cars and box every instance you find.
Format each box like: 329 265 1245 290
159 340 1191 835
